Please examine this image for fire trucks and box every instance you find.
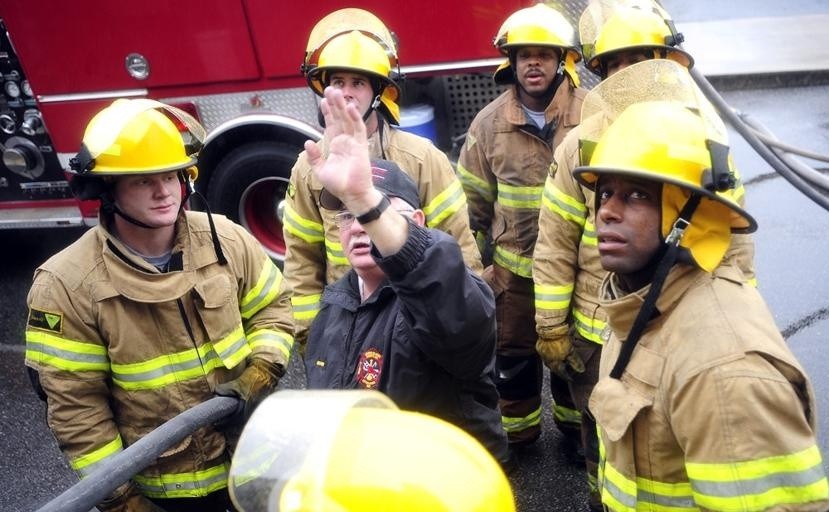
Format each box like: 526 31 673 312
1 0 603 273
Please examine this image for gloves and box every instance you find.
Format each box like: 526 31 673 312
532 322 586 390
213 357 284 423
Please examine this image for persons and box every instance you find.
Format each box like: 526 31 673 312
454 2 610 477
23 97 296 510
277 5 485 334
572 59 828 511
531 0 759 511
301 85 513 470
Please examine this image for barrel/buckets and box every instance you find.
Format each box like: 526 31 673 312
390 99 441 147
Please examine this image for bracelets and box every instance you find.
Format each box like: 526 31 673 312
356 190 393 225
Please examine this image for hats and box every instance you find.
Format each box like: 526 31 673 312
319 157 426 209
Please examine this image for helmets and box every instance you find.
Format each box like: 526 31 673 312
227 378 517 511
69 97 198 176
492 3 582 69
569 99 757 235
304 30 403 108
572 6 694 75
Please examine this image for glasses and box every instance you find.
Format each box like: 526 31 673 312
333 210 355 229
